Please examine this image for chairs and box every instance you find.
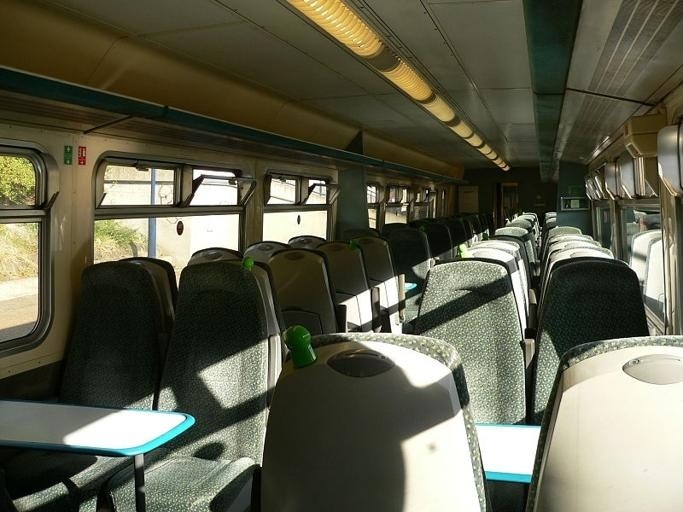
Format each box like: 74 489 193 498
0 207 680 512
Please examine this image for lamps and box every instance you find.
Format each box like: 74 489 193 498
463 132 484 147
475 142 510 172
446 114 472 138
280 1 386 60
376 54 435 102
418 92 456 124
